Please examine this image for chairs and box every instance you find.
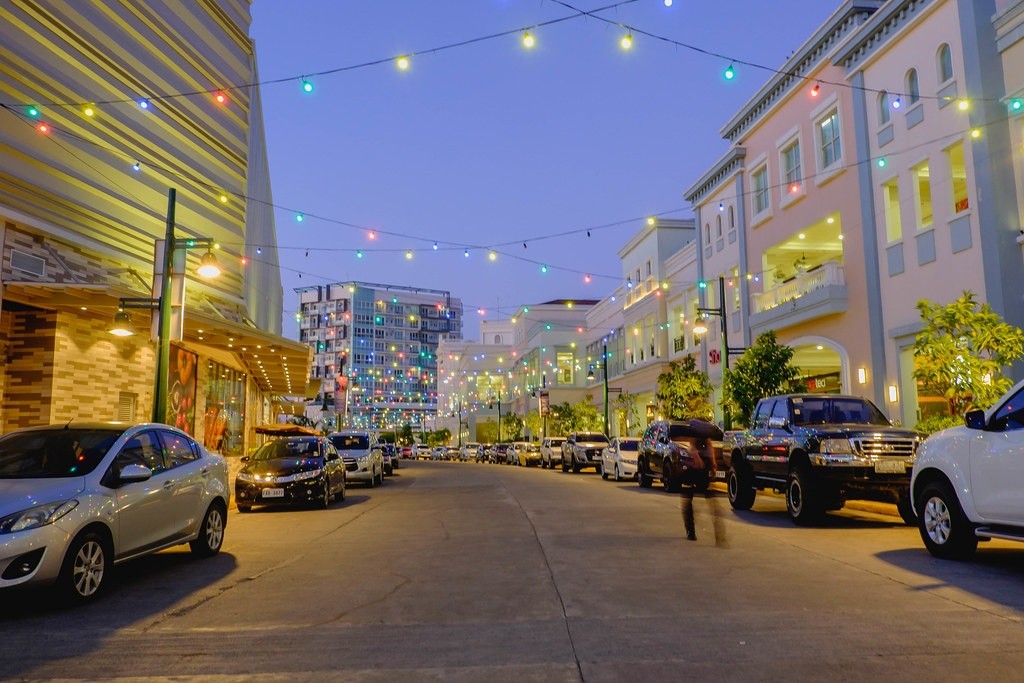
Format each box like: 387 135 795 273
308 441 318 452
810 410 826 424
359 438 368 446
831 412 847 423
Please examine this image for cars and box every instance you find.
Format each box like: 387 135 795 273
379 438 399 476
399 430 568 474
0 420 231 605
910 378 1024 561
235 433 347 513
600 436 641 482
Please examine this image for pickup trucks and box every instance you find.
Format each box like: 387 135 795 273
722 392 930 527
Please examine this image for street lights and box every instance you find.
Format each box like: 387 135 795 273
452 402 462 447
532 374 547 436
587 345 609 438
152 187 223 425
692 276 732 432
488 390 501 443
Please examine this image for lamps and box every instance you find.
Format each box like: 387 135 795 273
794 252 812 272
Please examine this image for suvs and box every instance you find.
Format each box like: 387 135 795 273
560 431 611 474
326 431 385 488
637 419 735 493
